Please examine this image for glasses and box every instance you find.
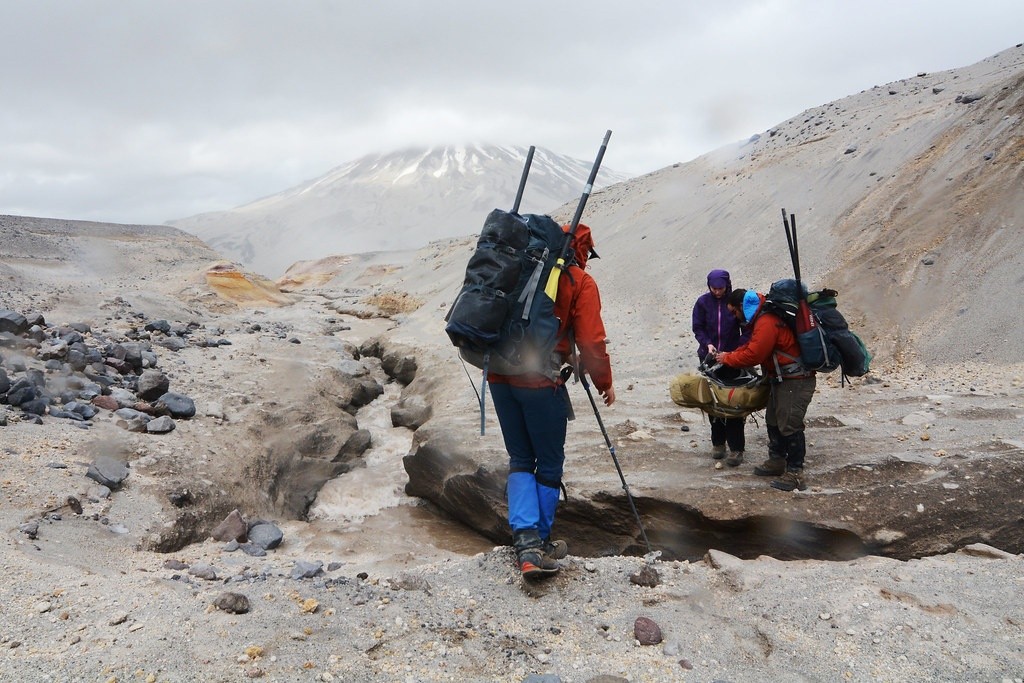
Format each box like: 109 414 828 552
725 304 740 317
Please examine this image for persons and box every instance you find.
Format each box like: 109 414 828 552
484 223 614 578
693 269 752 466
716 289 816 491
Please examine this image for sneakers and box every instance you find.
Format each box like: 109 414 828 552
517 548 560 581
753 450 787 476
725 450 743 465
541 537 568 560
710 444 726 458
769 466 808 490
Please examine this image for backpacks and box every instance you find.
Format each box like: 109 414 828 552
755 278 872 377
669 349 770 419
445 208 580 377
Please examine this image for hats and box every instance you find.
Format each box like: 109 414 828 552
589 244 600 260
